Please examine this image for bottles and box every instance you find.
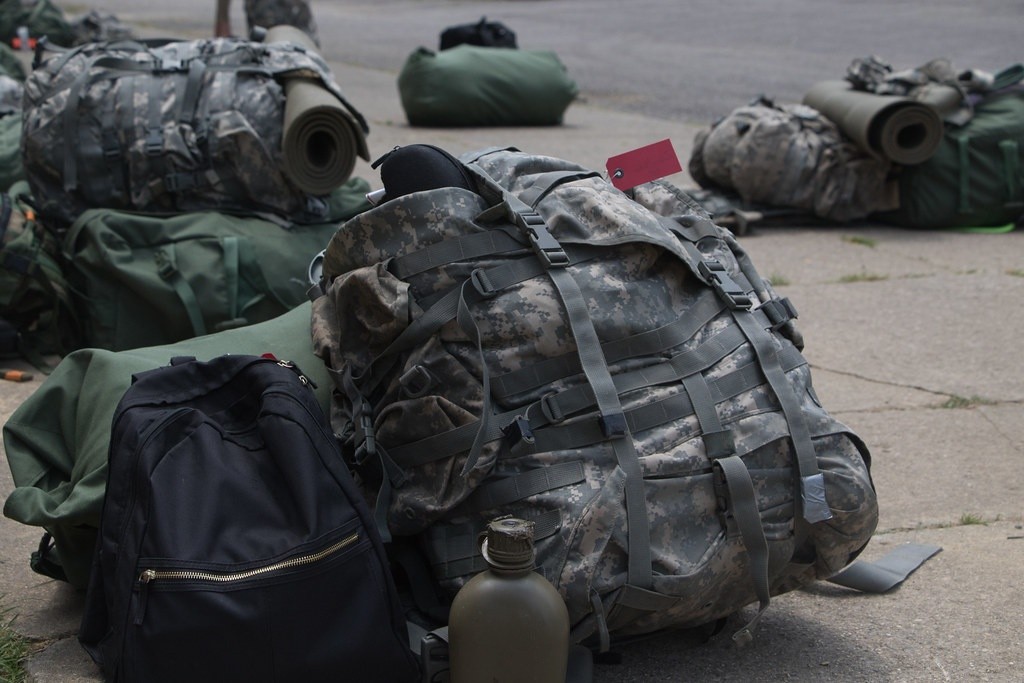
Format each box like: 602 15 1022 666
447 516 568 683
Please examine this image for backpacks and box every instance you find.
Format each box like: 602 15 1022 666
0 0 1024 683
